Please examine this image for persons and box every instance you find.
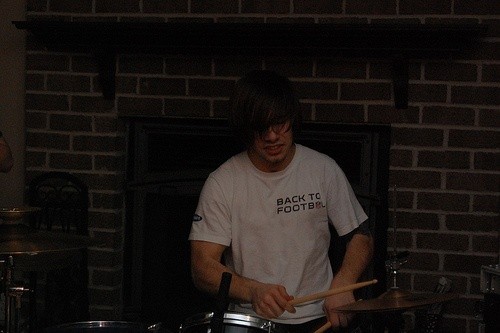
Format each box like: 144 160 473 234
188 73 375 333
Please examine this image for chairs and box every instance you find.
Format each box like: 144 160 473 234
0 172 89 333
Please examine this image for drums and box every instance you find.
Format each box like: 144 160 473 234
178 312 277 333
57 321 141 333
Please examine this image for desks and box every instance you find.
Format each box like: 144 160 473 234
1 231 94 333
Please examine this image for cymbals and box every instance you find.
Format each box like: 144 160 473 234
329 287 459 313
